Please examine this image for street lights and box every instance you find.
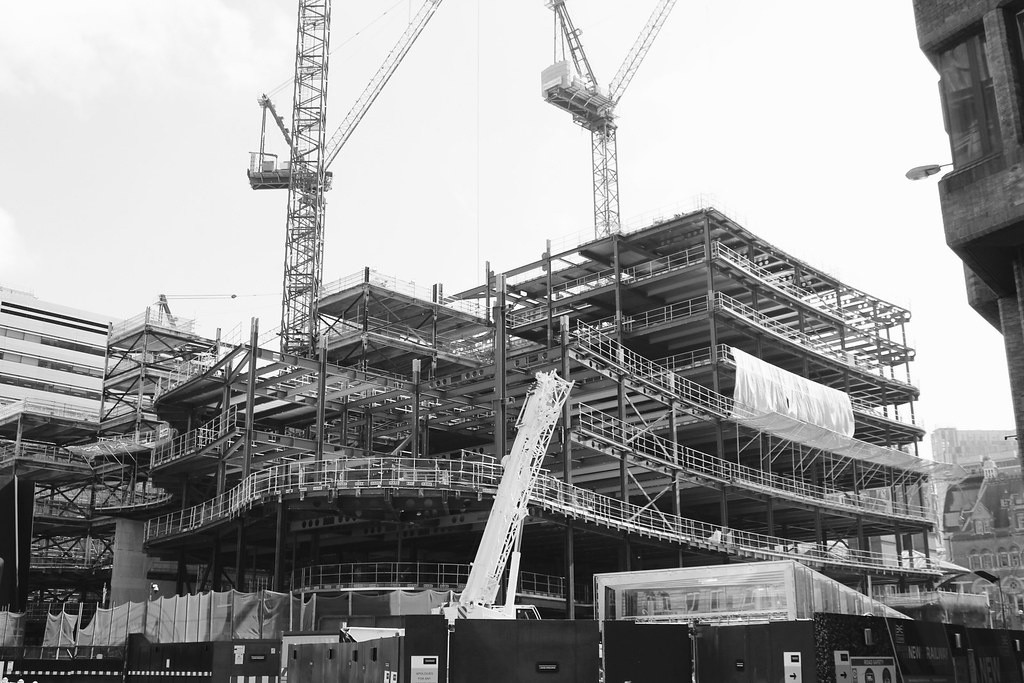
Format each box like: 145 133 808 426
974 568 1006 628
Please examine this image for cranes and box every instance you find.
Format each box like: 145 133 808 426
542 0 677 239
243 0 444 355
